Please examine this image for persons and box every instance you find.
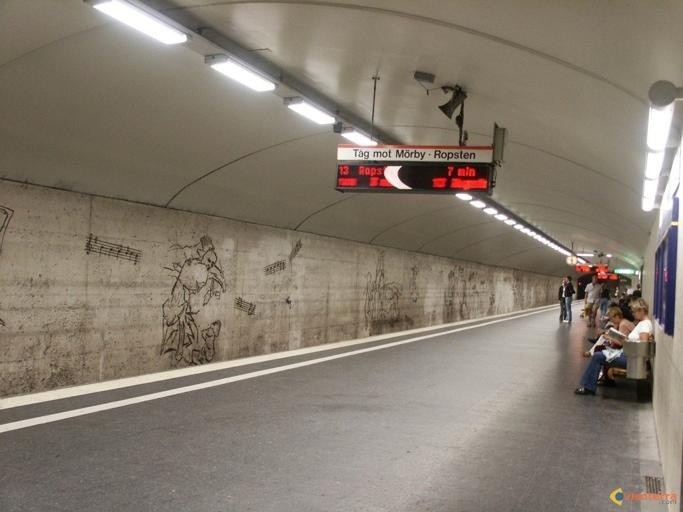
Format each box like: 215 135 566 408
557 273 654 395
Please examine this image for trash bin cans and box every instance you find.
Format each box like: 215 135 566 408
623 340 650 380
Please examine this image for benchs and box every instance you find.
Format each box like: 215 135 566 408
608 360 651 402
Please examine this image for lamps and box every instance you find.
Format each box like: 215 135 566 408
640 80 682 214
514 222 574 258
93 1 189 45
205 54 276 93
286 77 380 151
482 206 515 227
454 191 487 210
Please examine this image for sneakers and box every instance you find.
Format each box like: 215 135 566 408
559 319 571 324
575 387 596 396
583 351 592 357
587 323 597 328
587 338 598 344
